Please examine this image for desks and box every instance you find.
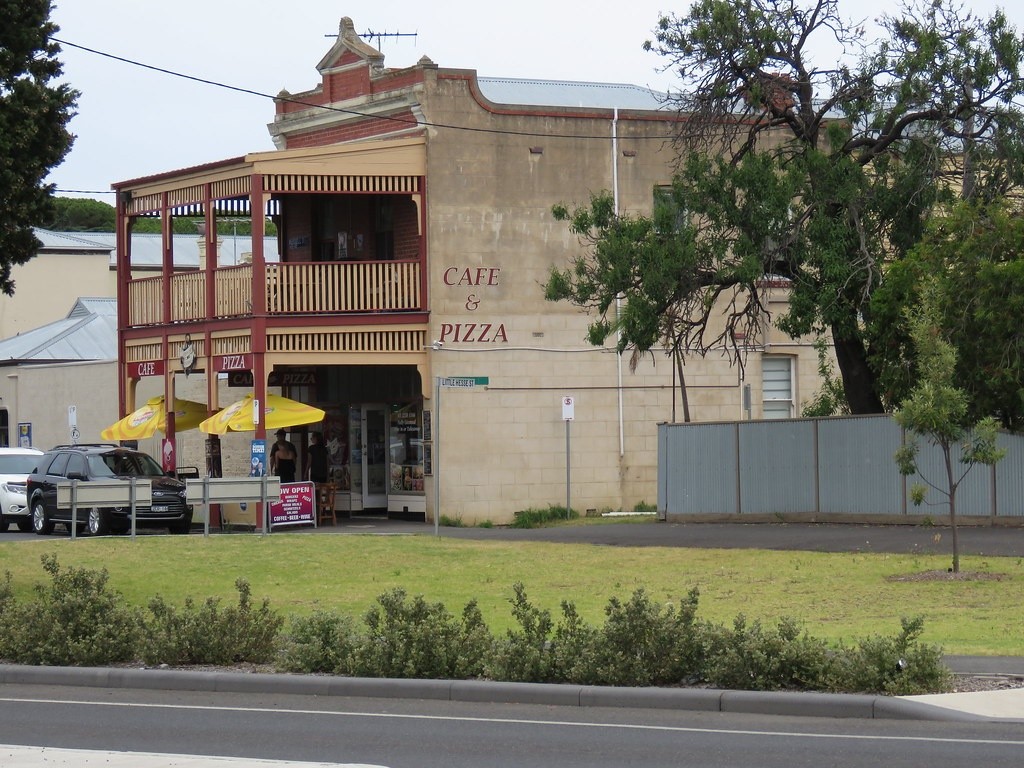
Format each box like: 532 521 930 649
269 282 323 310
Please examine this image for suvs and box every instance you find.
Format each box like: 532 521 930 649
26 443 194 535
390 441 424 478
0 447 47 532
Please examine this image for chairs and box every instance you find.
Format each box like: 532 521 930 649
315 482 338 527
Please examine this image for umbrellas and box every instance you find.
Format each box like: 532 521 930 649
199 390 326 434
101 394 225 441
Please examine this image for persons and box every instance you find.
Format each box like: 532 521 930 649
303 432 330 484
269 429 298 475
251 461 266 477
275 440 295 483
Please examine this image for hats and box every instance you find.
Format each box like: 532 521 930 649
274 429 286 436
